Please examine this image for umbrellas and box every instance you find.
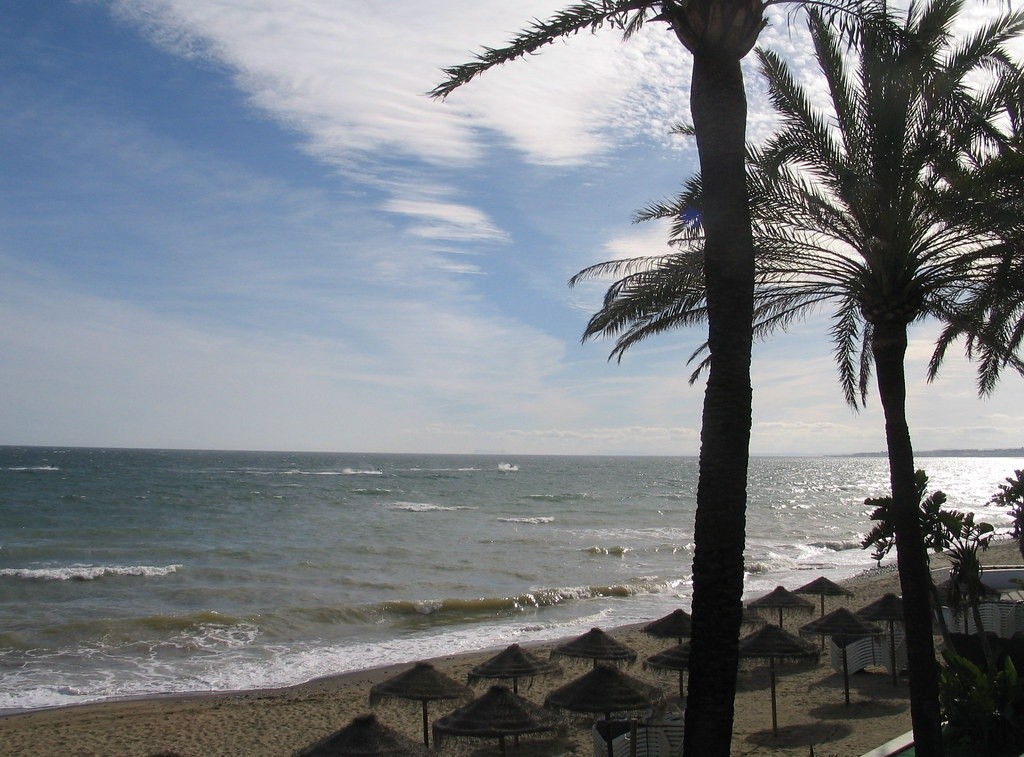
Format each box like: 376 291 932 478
743 604 767 624
638 607 692 697
738 622 817 735
291 711 442 756
643 640 691 671
466 641 564 694
431 683 565 756
796 606 885 704
934 573 1000 637
747 582 816 626
790 575 854 651
544 662 664 757
368 661 472 748
551 626 638 668
854 590 907 686
